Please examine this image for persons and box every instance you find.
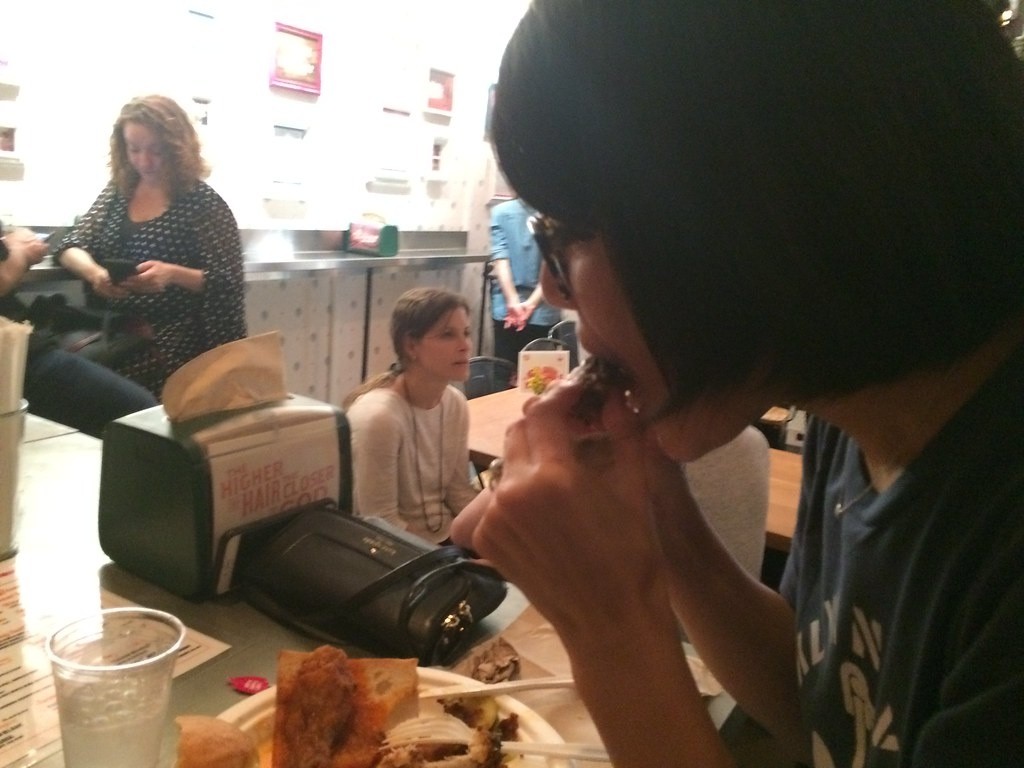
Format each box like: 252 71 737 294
0 219 159 441
55 95 247 404
451 0 1024 768
342 288 480 550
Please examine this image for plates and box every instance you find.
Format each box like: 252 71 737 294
211 654 581 768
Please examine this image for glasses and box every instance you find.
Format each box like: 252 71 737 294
524 206 617 302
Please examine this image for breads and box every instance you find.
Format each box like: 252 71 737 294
267 645 420 768
170 714 262 768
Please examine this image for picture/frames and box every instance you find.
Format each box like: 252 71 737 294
421 66 458 117
265 22 324 95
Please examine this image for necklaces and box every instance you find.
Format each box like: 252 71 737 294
403 373 444 533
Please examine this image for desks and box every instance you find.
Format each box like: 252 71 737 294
466 387 803 553
1 408 735 768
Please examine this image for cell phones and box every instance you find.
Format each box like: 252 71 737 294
101 259 138 286
42 231 57 243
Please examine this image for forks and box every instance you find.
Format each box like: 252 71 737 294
379 712 612 765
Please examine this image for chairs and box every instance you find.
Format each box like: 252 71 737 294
684 425 773 578
459 320 584 397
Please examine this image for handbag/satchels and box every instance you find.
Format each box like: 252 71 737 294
28 294 153 365
248 502 508 667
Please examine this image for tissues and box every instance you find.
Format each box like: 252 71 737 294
97 328 352 604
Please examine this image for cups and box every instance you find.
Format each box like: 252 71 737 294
48 606 186 768
0 398 30 561
